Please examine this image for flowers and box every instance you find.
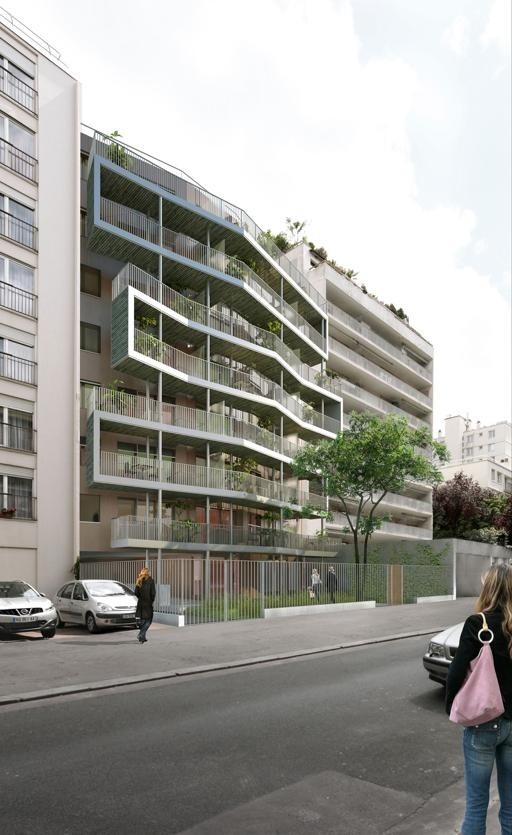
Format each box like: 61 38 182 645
1 505 17 513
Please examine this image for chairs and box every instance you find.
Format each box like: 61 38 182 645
124 462 158 480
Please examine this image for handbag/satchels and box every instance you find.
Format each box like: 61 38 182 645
449 612 504 727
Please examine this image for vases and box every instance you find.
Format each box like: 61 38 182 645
1 513 12 518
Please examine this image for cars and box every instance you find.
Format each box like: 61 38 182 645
0 580 57 639
421 618 466 686
53 579 139 634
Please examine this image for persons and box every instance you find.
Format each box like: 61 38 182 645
325 566 337 603
446 562 512 835
311 568 322 602
135 568 156 642
